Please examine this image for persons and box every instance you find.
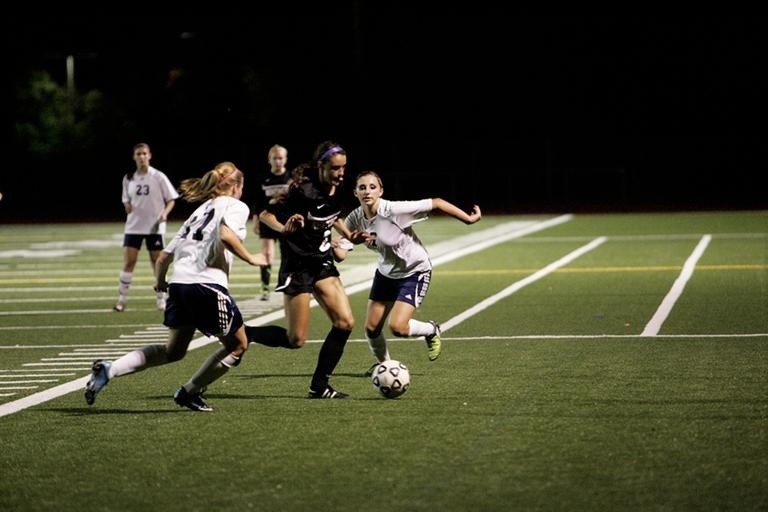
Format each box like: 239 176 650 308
240 141 375 399
251 143 307 302
113 143 177 312
332 169 483 378
85 161 269 414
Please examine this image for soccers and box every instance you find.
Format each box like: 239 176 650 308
371 360 410 398
